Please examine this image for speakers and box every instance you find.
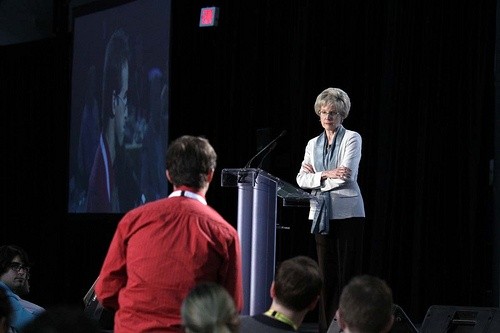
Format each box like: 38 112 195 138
82 275 116 331
327 304 419 333
419 305 500 333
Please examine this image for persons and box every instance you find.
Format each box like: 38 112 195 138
296 87 366 327
88 31 168 213
337 275 395 333
230 256 321 333
95 135 242 333
181 283 235 333
0 247 45 333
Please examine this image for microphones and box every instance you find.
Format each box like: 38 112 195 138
244 129 288 168
257 142 277 169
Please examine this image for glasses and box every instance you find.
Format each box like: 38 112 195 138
10 264 31 274
318 110 340 116
